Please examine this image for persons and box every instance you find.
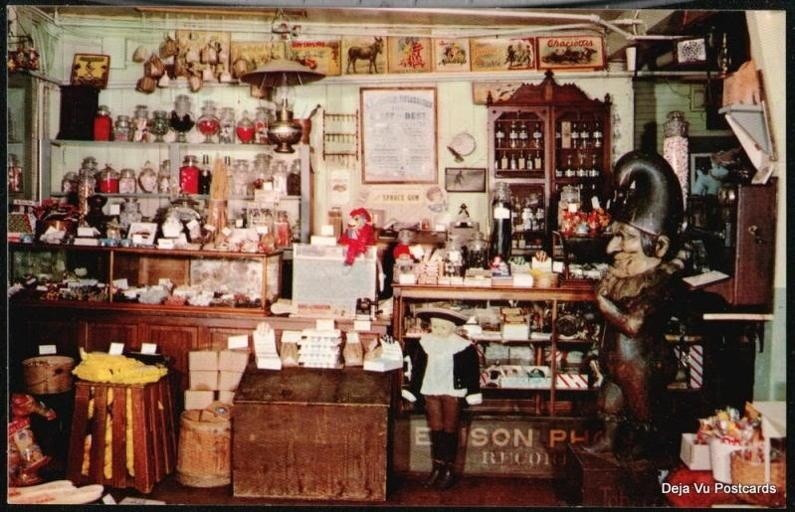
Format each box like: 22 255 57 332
583 148 687 472
403 302 487 492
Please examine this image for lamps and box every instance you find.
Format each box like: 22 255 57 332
240 9 326 151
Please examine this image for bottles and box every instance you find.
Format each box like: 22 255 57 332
463 231 492 269
495 119 605 243
7 107 24 193
488 182 513 266
61 99 302 253
329 209 343 242
398 266 417 286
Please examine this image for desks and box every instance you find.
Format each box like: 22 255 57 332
665 465 787 509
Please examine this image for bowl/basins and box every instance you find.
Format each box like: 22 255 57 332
606 59 627 72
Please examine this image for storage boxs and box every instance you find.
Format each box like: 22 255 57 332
230 367 390 503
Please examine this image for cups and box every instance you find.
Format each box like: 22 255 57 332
626 47 637 72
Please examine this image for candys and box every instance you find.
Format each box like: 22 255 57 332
697 407 761 461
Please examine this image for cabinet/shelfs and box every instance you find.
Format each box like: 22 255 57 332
50 138 315 306
10 313 300 389
392 283 598 419
488 72 614 258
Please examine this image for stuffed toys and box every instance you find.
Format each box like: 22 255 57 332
338 207 378 267
691 160 732 196
6 389 56 490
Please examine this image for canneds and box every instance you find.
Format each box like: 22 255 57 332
8 166 24 194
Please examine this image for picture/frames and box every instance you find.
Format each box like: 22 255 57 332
360 86 439 186
445 168 486 193
